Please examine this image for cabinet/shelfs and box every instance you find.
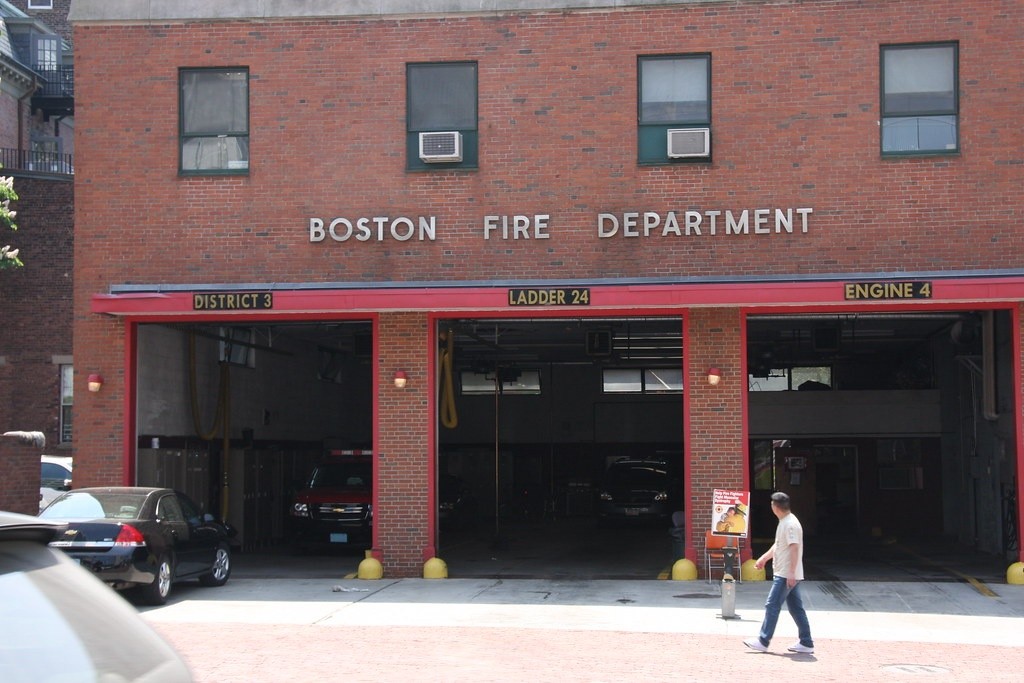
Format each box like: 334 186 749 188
137 447 307 552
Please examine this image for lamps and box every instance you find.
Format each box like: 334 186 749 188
391 370 408 388
749 358 773 380
86 374 104 392
498 367 522 383
470 360 496 375
706 368 722 386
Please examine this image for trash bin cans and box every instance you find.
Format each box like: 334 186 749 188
669 511 684 566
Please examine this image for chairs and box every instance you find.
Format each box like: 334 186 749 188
704 530 742 584
113 506 138 519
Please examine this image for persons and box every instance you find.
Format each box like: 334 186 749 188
741 492 814 653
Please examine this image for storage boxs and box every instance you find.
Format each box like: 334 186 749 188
227 160 249 170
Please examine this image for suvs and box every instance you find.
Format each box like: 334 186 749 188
594 456 682 532
289 449 373 547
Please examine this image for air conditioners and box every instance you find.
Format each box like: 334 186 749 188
418 131 463 164
666 128 710 159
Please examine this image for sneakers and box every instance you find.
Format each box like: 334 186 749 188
743 637 769 653
787 642 815 653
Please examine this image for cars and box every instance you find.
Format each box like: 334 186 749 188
33 485 234 606
40 453 73 510
0 520 194 682
